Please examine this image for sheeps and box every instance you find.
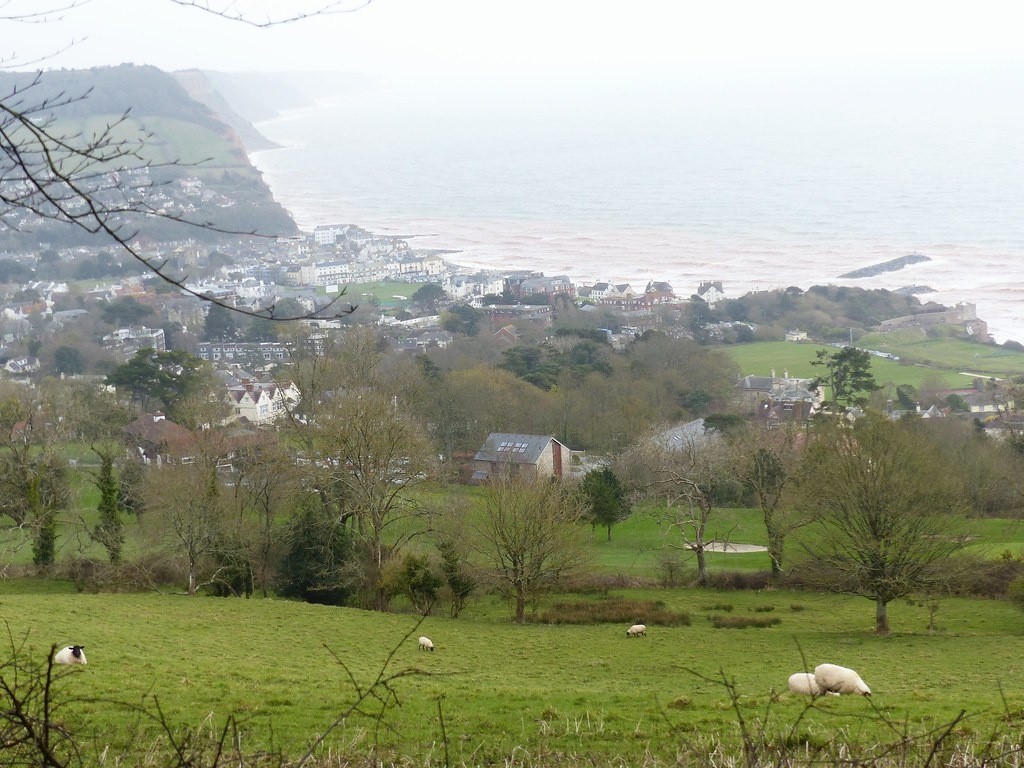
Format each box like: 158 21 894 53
788 673 824 695
814 663 872 696
626 625 646 637
55 646 88 665
419 636 434 651
